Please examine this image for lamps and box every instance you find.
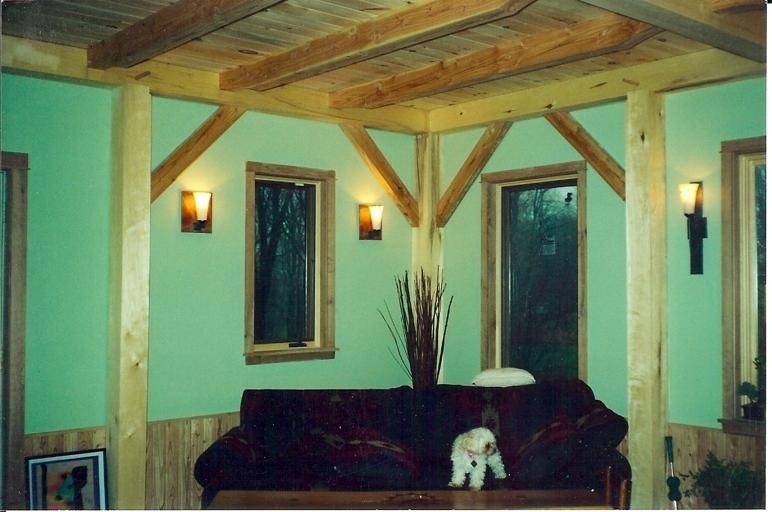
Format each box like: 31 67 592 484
368 205 385 239
191 191 213 230
677 181 700 240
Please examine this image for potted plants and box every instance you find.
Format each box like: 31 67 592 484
736 351 766 420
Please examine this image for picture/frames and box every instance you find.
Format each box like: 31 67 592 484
25 447 109 510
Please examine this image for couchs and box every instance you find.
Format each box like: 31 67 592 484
195 379 633 510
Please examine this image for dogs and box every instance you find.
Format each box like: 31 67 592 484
448 426 507 492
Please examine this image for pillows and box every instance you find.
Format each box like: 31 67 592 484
332 425 423 482
509 410 576 481
